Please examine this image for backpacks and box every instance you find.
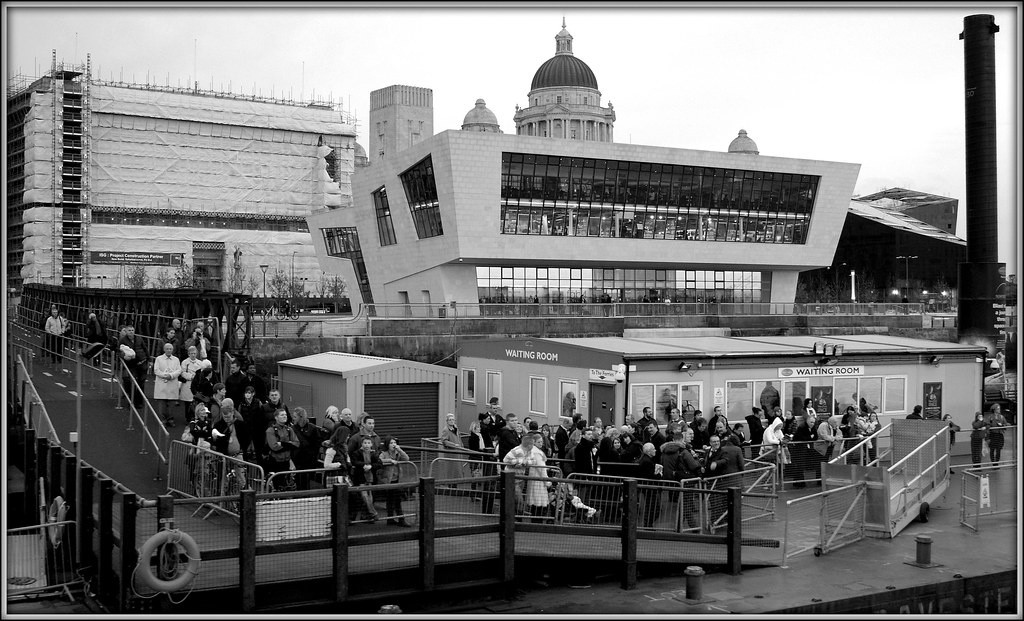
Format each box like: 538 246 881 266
262 425 291 459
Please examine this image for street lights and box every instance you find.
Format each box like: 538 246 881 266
836 263 847 303
97 276 106 288
75 342 107 565
896 255 919 298
1009 274 1016 326
260 265 269 320
300 278 308 309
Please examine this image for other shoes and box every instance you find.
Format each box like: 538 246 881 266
950 470 955 474
162 420 167 428
397 520 412 527
168 419 176 428
386 519 398 526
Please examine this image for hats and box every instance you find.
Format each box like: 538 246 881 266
44 308 51 312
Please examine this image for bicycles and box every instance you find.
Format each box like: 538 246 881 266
193 450 254 528
276 306 299 320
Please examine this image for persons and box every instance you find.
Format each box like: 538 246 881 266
970 412 989 472
38 305 71 363
86 313 106 367
746 398 881 488
906 405 923 419
118 318 412 528
442 398 749 534
942 414 961 474
986 403 1007 469
576 293 717 315
479 295 540 317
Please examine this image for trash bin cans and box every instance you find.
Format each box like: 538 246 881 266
144 374 156 399
439 308 446 318
325 303 335 313
1001 409 1014 424
868 306 874 315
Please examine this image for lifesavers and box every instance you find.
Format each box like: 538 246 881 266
921 501 930 521
134 529 202 593
46 495 67 544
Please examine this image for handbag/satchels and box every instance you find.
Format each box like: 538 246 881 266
813 439 827 455
